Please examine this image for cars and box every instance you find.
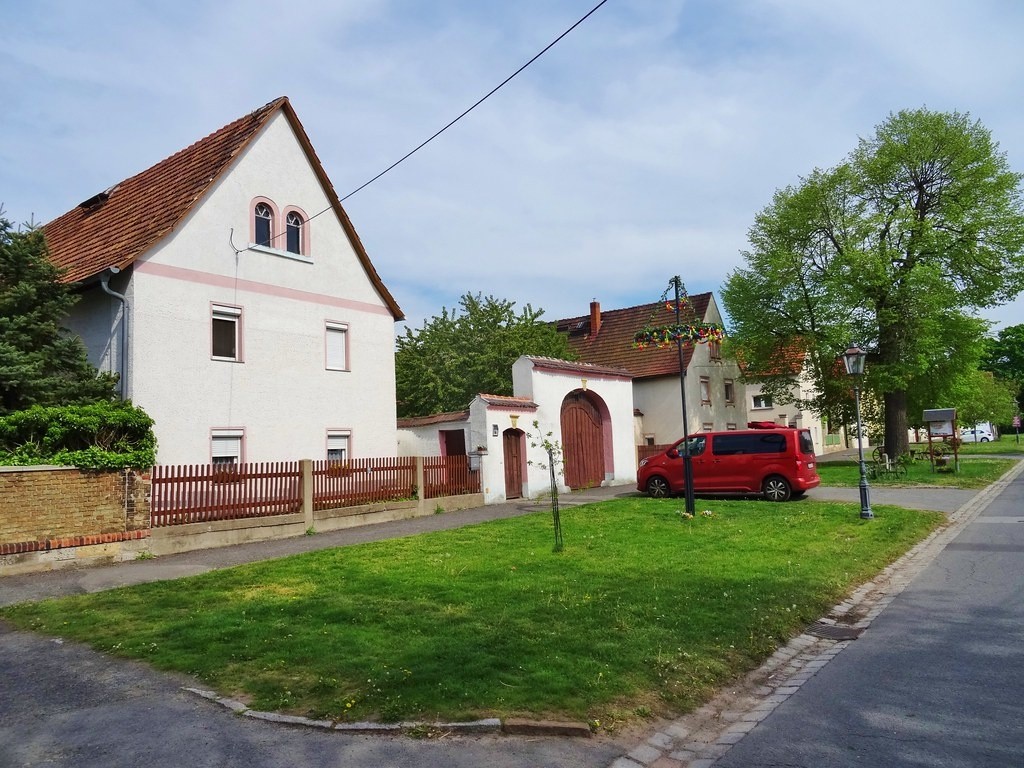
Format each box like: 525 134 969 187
954 428 994 442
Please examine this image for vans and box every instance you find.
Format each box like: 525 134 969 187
636 427 821 501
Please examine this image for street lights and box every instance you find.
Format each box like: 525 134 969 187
1012 398 1021 445
840 340 875 520
